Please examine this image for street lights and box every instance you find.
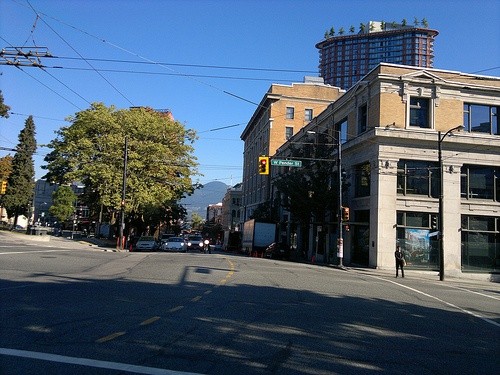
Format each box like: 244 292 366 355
438 124 466 280
307 130 345 270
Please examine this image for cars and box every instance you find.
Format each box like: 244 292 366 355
163 237 188 252
136 236 159 252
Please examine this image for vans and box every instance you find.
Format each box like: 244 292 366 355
159 234 175 251
187 234 204 252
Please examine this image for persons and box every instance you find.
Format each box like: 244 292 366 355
86 229 91 239
394 246 406 279
202 232 212 254
216 233 222 244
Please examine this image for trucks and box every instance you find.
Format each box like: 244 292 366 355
241 219 277 256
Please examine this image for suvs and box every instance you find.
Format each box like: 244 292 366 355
264 242 290 260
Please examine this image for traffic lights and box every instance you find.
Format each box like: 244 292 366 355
0 181 9 195
258 156 269 175
343 207 349 221
